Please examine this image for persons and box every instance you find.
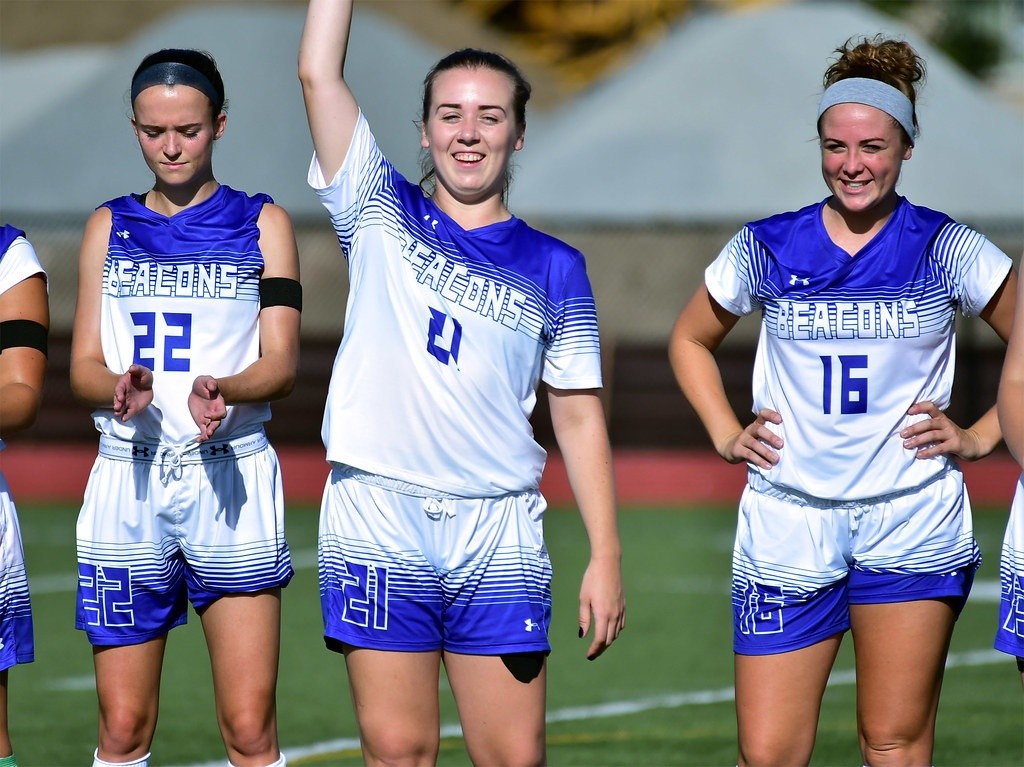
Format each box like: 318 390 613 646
994 251 1024 684
68 49 302 767
296 0 625 767
668 39 1017 767
0 224 50 767
